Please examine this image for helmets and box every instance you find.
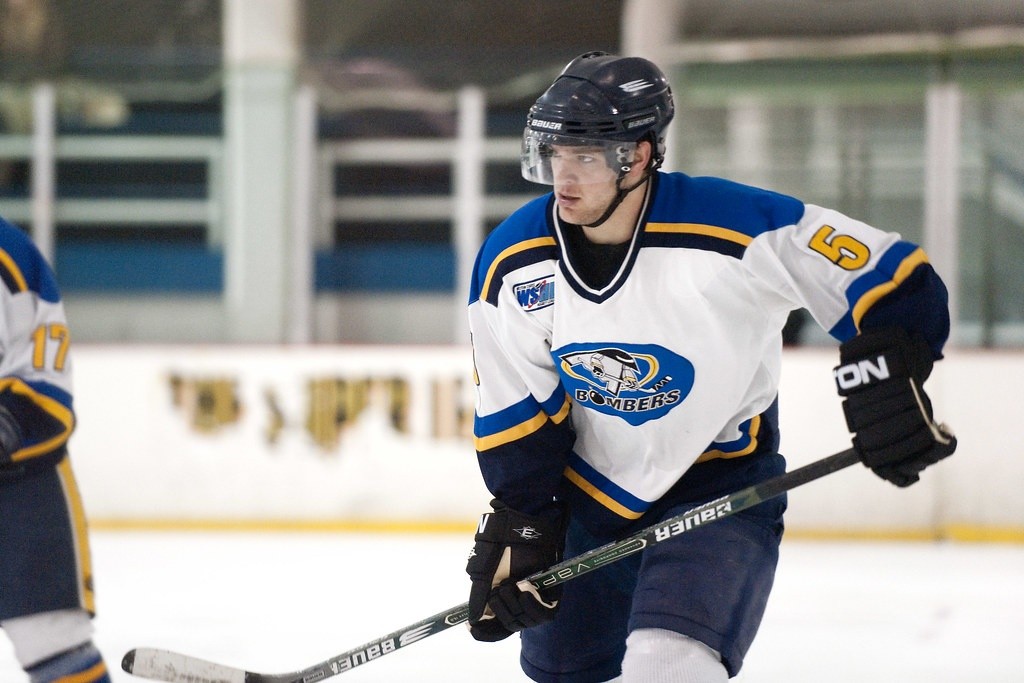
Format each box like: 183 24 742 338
526 49 676 147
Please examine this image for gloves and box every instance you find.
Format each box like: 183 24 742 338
467 513 563 642
835 322 956 487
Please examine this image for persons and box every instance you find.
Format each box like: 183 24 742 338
0 213 112 683
466 53 957 683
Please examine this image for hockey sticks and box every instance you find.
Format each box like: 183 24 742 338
122 446 863 683
